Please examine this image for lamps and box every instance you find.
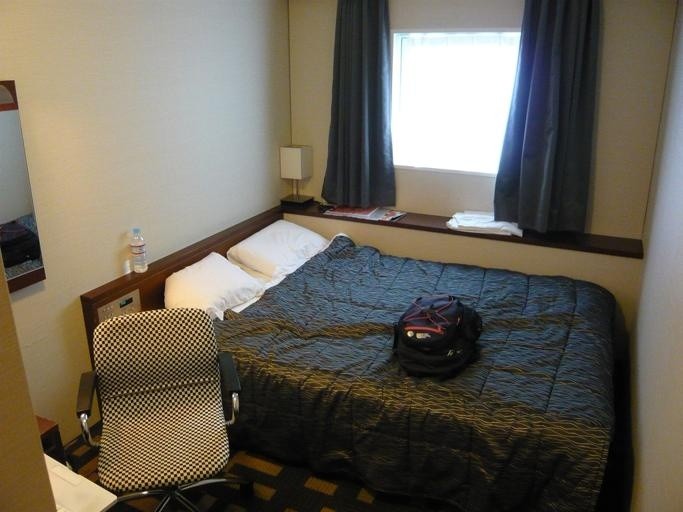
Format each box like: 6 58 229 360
280 143 314 207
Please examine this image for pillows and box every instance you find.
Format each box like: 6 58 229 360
163 252 263 322
227 218 331 282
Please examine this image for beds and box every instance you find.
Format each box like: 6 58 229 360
80 200 634 512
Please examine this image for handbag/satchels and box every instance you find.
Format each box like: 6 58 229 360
393 295 482 377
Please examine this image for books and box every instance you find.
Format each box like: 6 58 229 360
322 204 408 223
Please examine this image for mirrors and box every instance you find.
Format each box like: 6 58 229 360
0 80 46 294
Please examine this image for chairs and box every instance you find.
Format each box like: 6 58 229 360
75 307 241 512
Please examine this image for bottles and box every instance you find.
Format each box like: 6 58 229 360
129 227 149 275
